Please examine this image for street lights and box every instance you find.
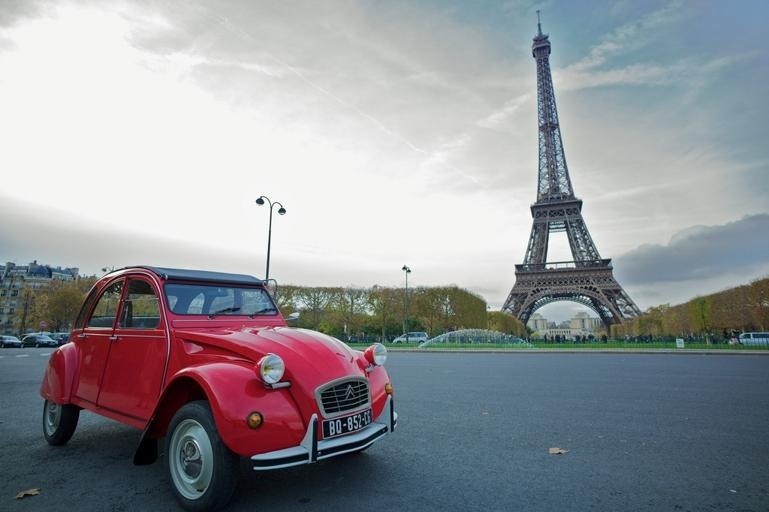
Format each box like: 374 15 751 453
254 193 287 290
102 263 115 318
399 264 412 346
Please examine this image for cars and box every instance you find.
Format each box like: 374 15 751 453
33 262 403 512
0 322 73 347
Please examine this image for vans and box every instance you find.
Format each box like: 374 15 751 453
727 330 769 348
391 328 429 345
